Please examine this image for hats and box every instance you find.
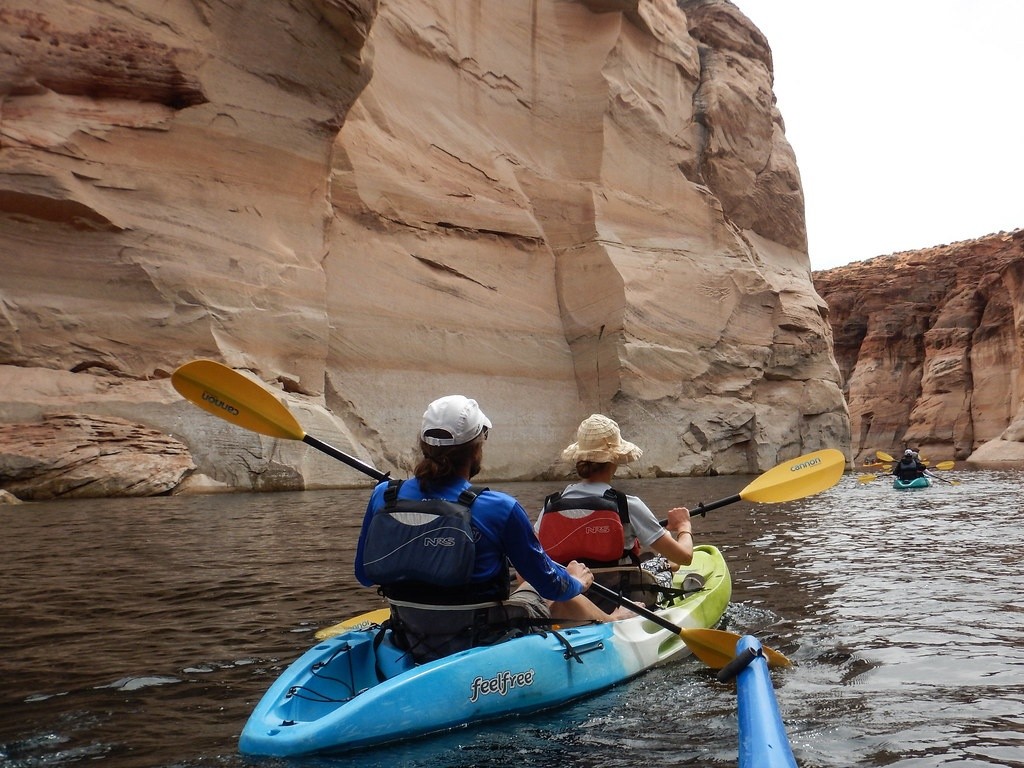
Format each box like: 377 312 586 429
904 449 913 456
560 412 644 469
418 393 494 443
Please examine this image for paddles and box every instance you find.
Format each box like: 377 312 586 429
882 461 930 469
858 460 955 483
876 450 959 485
169 357 793 672
314 446 846 641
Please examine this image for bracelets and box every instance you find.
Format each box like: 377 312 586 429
677 531 694 540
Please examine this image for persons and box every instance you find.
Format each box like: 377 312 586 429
893 448 926 480
534 414 693 607
354 394 645 664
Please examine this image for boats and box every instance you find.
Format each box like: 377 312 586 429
892 475 933 489
862 461 884 468
231 543 733 760
